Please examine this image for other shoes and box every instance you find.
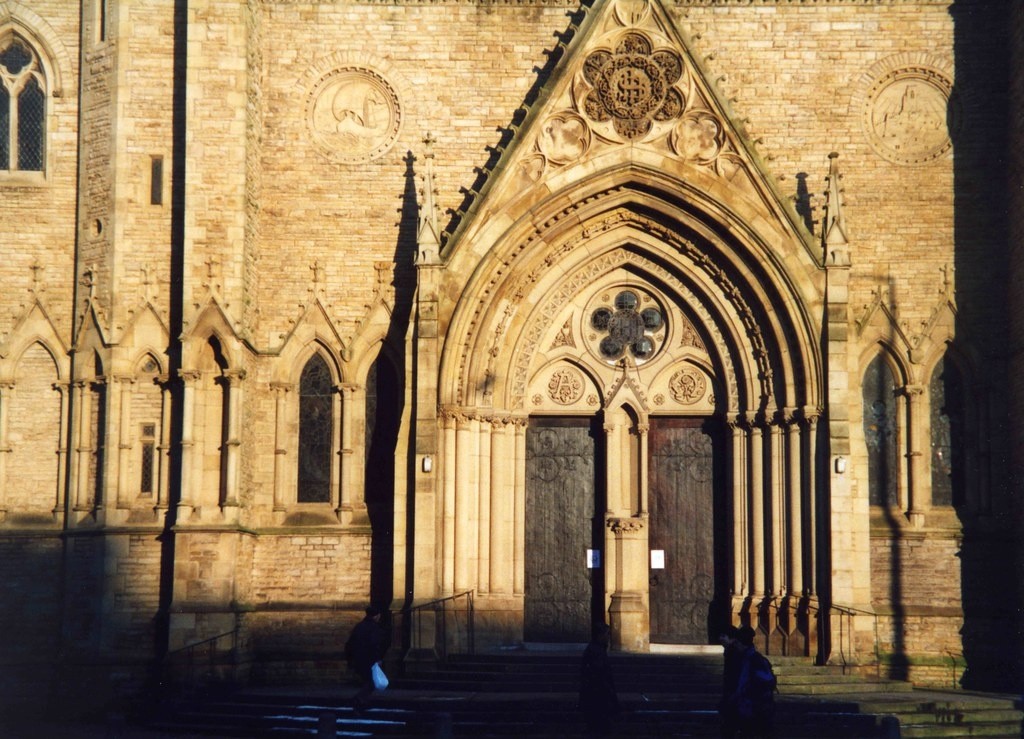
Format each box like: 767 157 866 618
350 696 363 716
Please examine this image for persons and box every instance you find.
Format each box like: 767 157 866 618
581 624 622 739
719 622 776 739
341 606 387 716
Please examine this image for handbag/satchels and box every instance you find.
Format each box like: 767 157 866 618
371 662 389 695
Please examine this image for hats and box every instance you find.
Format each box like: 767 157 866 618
734 627 754 645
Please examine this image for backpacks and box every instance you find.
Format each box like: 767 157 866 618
741 651 776 690
